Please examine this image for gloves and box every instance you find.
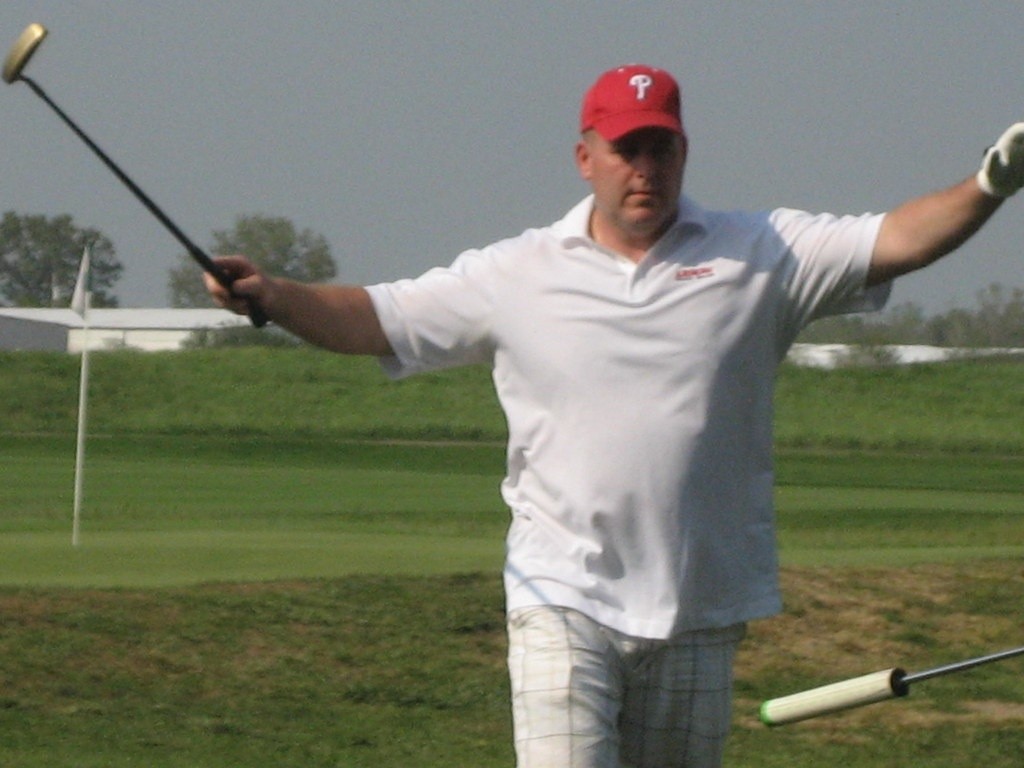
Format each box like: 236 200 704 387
975 120 1024 199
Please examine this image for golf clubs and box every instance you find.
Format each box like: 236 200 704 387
1 20 274 332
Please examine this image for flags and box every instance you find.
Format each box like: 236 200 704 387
68 249 94 320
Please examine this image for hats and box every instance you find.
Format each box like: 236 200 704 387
579 64 684 143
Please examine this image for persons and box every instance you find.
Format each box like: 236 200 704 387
203 68 1024 768
